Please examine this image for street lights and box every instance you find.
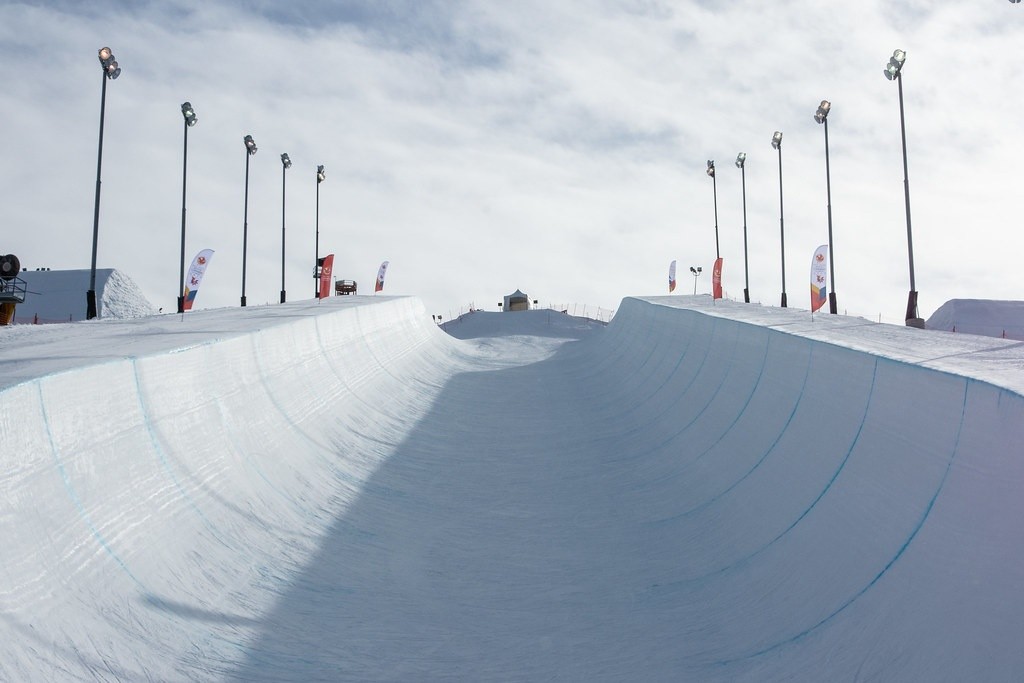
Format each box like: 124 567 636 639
883 49 926 329
690 266 702 294
279 152 292 303
241 134 258 306
706 159 722 297
178 101 198 312
814 100 838 314
315 164 325 297
736 152 751 303
86 46 122 319
771 131 788 307
